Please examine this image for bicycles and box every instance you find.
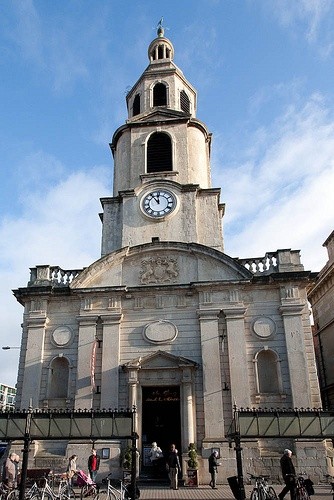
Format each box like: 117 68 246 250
246 472 278 500
286 473 312 500
0 474 76 500
92 472 132 500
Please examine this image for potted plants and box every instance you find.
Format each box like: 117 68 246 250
187 443 200 487
123 450 132 489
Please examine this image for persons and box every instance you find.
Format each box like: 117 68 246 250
208 451 222 489
165 444 182 490
150 442 163 477
88 448 100 483
3 453 20 492
278 448 297 500
66 454 78 486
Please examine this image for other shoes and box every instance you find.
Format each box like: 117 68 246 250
212 487 218 489
208 484 213 487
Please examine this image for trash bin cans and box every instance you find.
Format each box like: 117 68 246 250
226 476 240 498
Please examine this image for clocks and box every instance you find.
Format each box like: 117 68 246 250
142 190 176 218
